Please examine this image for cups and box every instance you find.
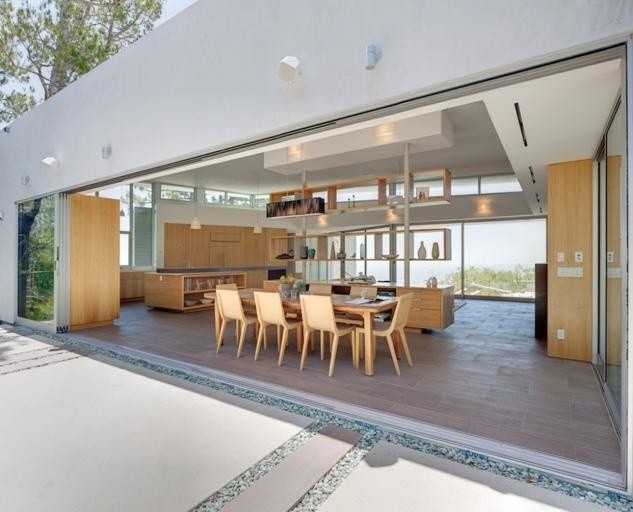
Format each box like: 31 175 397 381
361 290 367 300
184 277 236 290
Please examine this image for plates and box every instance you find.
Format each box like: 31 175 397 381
383 254 399 259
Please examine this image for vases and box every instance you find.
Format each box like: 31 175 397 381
306 248 315 259
430 242 440 259
358 242 365 259
416 242 427 259
329 241 335 258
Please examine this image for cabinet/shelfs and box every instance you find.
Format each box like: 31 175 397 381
267 165 453 212
533 262 547 339
140 269 248 316
313 278 457 337
268 226 452 264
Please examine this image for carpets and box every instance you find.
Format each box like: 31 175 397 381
453 301 467 313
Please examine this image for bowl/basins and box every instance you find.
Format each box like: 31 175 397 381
185 299 212 305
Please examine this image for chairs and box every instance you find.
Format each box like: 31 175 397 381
200 272 416 379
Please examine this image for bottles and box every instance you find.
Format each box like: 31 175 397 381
306 248 316 259
347 193 358 209
417 240 426 259
432 242 439 259
328 241 335 260
337 251 346 259
427 277 437 287
359 243 364 259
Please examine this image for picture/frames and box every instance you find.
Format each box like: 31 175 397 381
415 185 429 203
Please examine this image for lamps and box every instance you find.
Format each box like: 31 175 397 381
250 174 264 236
21 175 28 186
186 167 206 231
364 47 381 70
0 211 5 222
100 145 109 160
41 157 57 170
265 146 326 220
277 55 302 83
